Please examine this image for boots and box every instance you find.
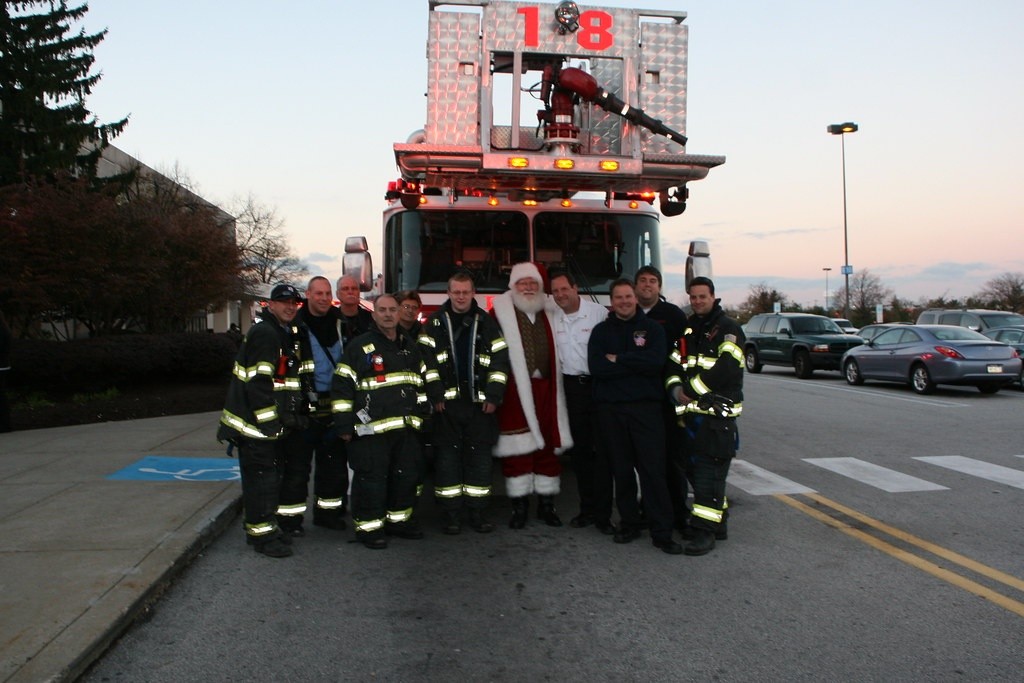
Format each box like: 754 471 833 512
533 473 562 527
504 472 533 529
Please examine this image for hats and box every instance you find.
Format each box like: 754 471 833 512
509 261 548 296
271 285 302 301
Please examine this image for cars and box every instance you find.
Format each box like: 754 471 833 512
840 325 1022 395
853 324 905 340
887 322 914 326
829 317 860 335
979 325 1024 384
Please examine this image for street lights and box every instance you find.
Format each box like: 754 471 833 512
823 268 832 310
827 122 857 319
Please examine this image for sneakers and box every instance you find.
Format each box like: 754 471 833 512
613 526 641 543
278 523 305 537
254 538 292 557
383 522 422 540
685 531 716 555
247 532 292 544
313 513 346 530
653 536 682 554
467 516 492 533
594 519 616 534
355 530 387 548
674 520 690 530
569 508 594 527
679 524 728 540
439 512 460 534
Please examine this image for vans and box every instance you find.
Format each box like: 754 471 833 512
915 307 1024 332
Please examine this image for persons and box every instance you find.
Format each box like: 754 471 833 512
587 267 745 555
489 263 575 530
420 273 509 535
543 269 618 535
217 273 423 559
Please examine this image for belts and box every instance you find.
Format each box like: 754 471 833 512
563 376 591 384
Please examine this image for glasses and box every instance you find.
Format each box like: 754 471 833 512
450 290 474 296
400 304 419 313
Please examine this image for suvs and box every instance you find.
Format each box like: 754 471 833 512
744 312 863 379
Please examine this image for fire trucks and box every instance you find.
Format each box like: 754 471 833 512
339 0 728 318
